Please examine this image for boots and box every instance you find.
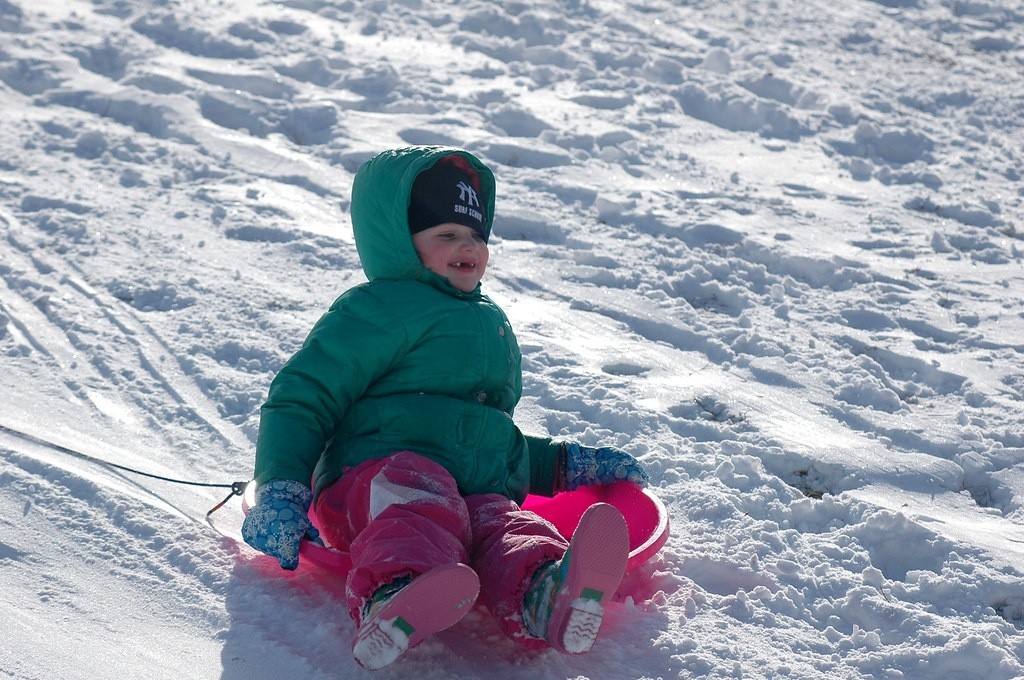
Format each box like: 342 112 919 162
525 503 630 657
354 560 478 668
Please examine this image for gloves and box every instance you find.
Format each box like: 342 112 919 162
242 479 319 571
565 438 649 488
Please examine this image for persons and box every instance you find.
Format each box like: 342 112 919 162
241 144 651 671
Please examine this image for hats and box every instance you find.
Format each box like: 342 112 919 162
407 158 489 246
